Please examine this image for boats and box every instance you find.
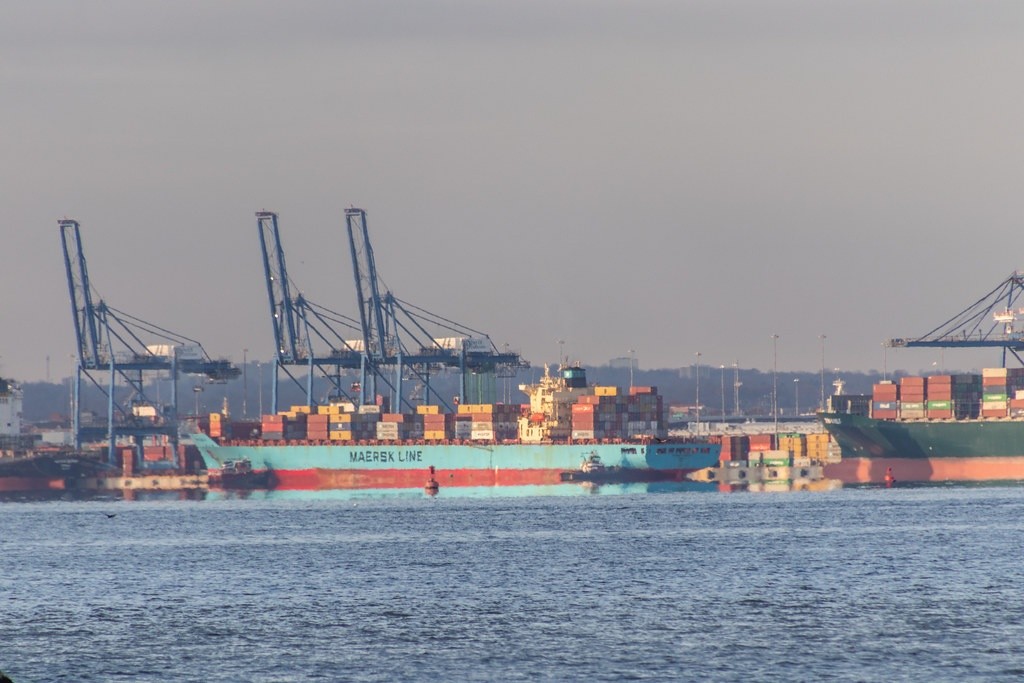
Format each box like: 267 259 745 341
186 366 727 493
814 363 1024 483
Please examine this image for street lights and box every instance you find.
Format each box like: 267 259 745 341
695 352 703 436
770 333 780 451
256 364 262 422
627 349 635 388
557 339 566 367
720 365 725 423
817 334 827 413
793 378 800 416
242 348 249 418
